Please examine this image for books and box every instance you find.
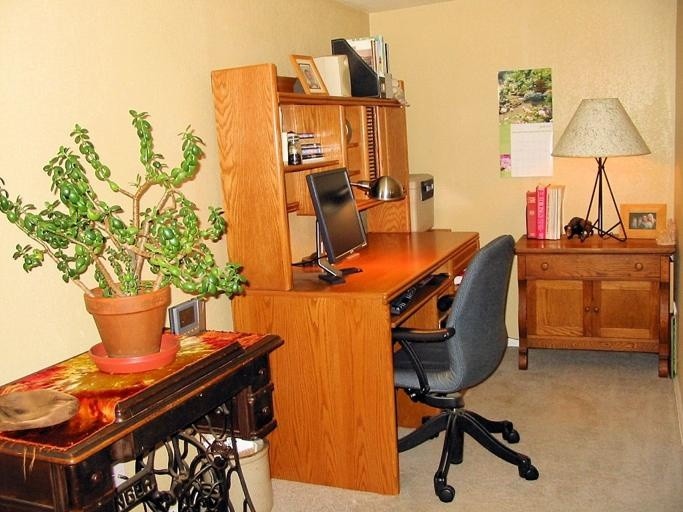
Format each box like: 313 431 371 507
299 132 325 164
348 34 389 75
524 183 565 241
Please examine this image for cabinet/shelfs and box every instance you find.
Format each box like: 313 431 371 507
211 61 376 289
513 231 675 378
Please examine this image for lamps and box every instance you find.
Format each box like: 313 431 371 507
314 173 404 277
552 97 652 241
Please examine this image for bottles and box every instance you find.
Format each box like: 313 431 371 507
288 135 303 167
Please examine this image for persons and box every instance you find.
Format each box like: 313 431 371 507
647 214 656 225
640 215 652 229
304 68 320 89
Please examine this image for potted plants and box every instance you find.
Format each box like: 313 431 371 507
0 109 247 355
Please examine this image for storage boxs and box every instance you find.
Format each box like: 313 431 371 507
379 101 407 195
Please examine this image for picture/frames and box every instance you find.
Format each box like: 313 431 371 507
289 54 329 95
618 202 667 238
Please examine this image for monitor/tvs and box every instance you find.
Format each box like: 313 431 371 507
305 166 368 285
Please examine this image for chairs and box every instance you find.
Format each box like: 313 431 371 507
397 232 539 502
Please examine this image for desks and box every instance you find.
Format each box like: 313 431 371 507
0 330 285 512
229 232 481 495
